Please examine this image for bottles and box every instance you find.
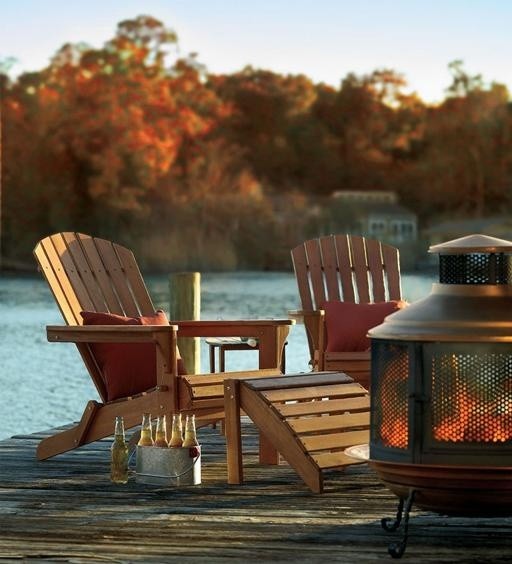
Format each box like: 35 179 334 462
109 410 199 485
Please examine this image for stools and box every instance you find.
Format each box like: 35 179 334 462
204 338 288 436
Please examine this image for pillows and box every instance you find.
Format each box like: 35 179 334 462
322 299 408 350
78 309 188 403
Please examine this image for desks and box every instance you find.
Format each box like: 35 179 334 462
171 316 295 465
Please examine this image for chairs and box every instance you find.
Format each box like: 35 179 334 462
288 233 412 473
33 230 371 494
